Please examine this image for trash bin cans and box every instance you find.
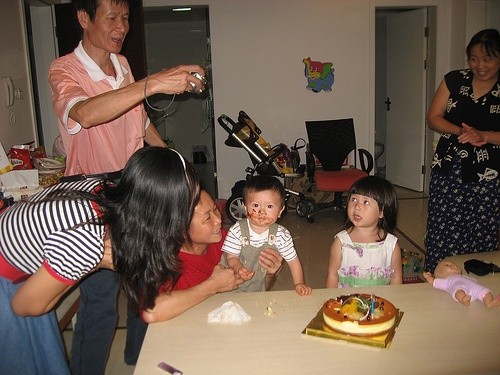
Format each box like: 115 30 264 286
192 145 208 164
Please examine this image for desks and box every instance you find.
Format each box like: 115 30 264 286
132 282 500 375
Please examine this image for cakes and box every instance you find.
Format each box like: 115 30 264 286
322 294 397 337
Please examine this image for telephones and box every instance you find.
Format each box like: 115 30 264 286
3 75 17 126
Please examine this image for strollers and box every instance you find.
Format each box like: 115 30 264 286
218 110 314 222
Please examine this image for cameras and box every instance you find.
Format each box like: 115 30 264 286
189 72 207 95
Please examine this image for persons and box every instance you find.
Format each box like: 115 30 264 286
219 176 312 296
48 0 208 375
0 145 199 375
138 190 284 324
423 260 498 309
326 175 402 289
424 28 500 278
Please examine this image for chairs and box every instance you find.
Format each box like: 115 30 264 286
305 118 374 223
54 286 81 333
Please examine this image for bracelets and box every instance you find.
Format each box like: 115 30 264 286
460 128 462 135
